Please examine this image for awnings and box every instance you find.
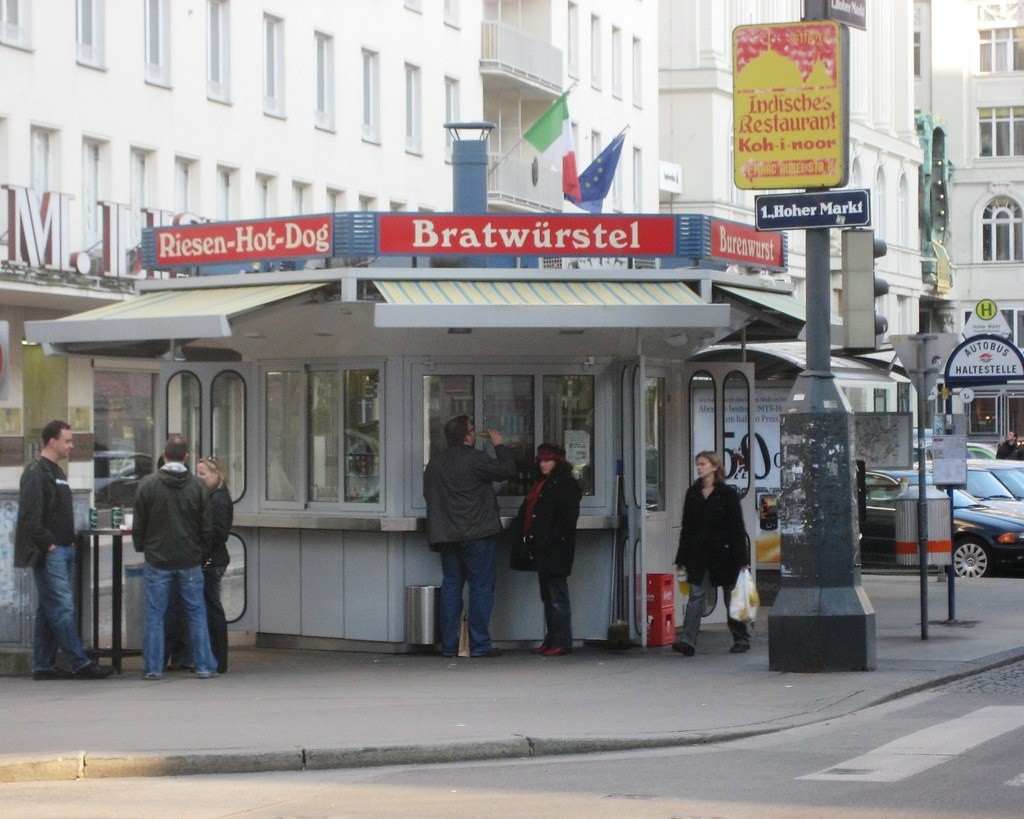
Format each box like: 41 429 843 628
23 281 334 344
372 279 731 328
712 283 844 346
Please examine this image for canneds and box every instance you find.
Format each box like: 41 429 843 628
111 506 123 530
89 507 99 529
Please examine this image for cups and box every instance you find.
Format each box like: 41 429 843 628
124 514 133 529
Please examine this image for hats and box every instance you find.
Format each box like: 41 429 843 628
534 442 565 461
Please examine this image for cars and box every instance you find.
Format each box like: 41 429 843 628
913 459 1024 516
864 469 1024 578
94 451 153 507
913 441 997 464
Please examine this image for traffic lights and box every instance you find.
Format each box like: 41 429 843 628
841 229 889 354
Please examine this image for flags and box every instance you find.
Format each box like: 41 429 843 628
564 134 625 213
523 91 581 203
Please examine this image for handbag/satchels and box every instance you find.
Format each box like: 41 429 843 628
730 568 759 620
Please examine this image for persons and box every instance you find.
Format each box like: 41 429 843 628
672 451 752 655
510 442 583 656
197 457 234 674
132 436 220 680
996 432 1018 459
422 415 518 658
13 420 114 680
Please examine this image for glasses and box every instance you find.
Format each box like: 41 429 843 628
207 456 218 471
466 426 476 433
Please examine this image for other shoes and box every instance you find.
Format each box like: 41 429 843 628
75 662 113 678
532 643 549 653
544 646 570 655
731 644 750 652
143 660 225 679
672 641 696 656
473 647 501 656
32 667 73 680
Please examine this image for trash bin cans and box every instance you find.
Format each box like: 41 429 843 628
895 489 954 566
407 584 444 644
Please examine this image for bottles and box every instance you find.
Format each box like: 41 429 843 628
474 429 502 438
505 471 535 496
